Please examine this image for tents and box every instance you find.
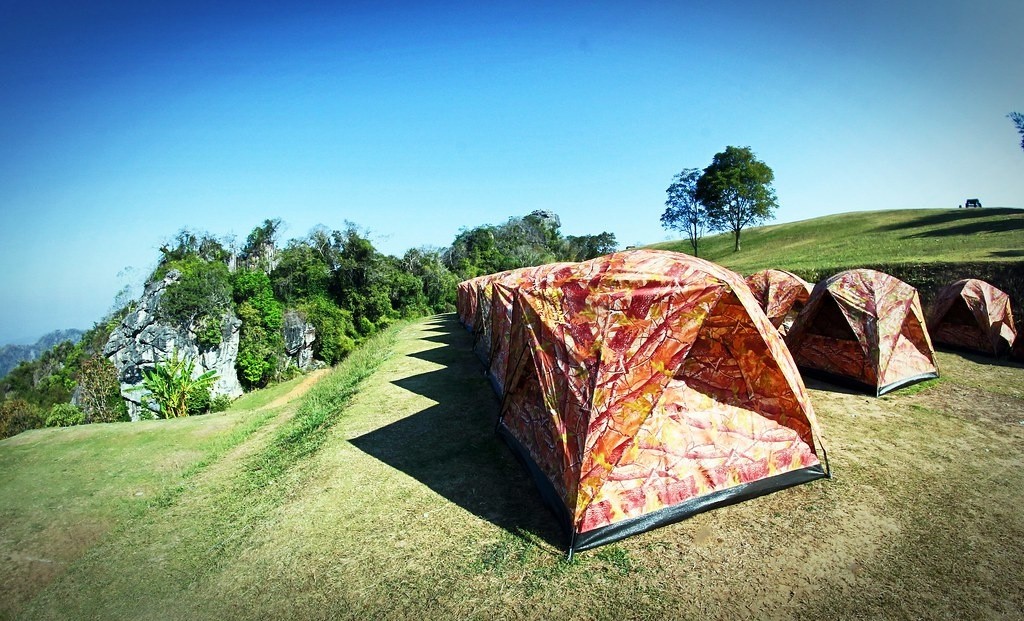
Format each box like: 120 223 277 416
929 278 1017 365
455 247 830 563
746 269 815 338
784 268 940 397
